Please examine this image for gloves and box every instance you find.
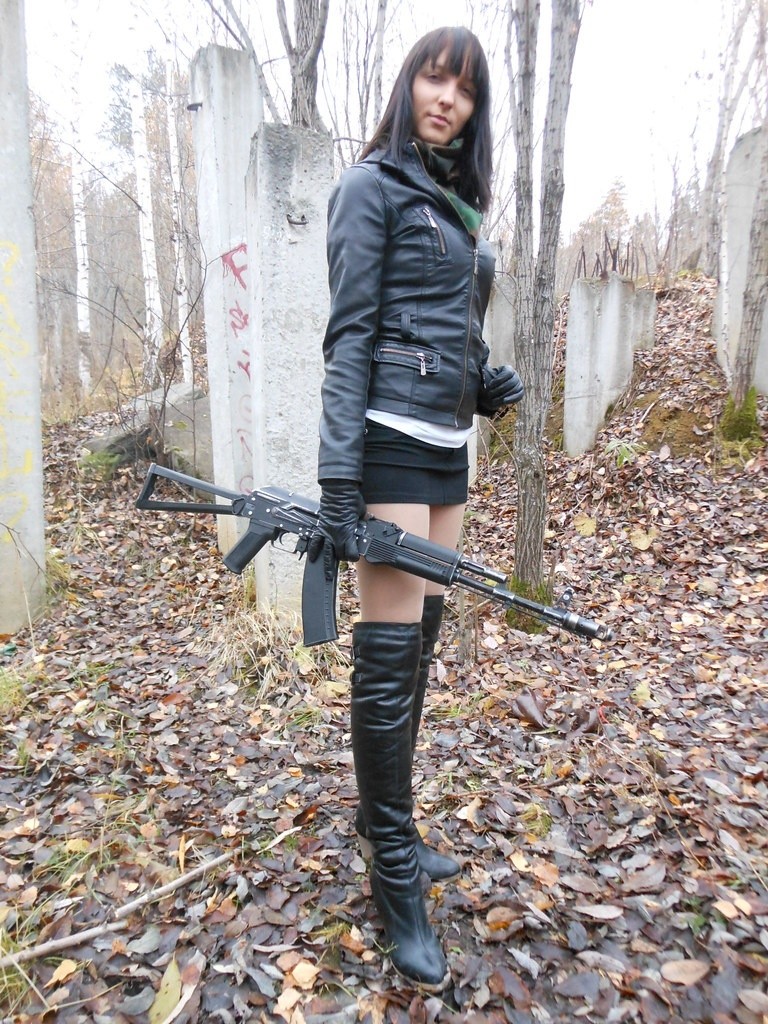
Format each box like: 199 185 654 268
483 364 525 410
308 479 374 581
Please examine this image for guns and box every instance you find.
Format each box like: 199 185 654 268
134 460 608 649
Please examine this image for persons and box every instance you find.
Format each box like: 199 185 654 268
307 26 525 994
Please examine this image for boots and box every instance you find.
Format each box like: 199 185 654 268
354 594 462 879
349 622 450 992
